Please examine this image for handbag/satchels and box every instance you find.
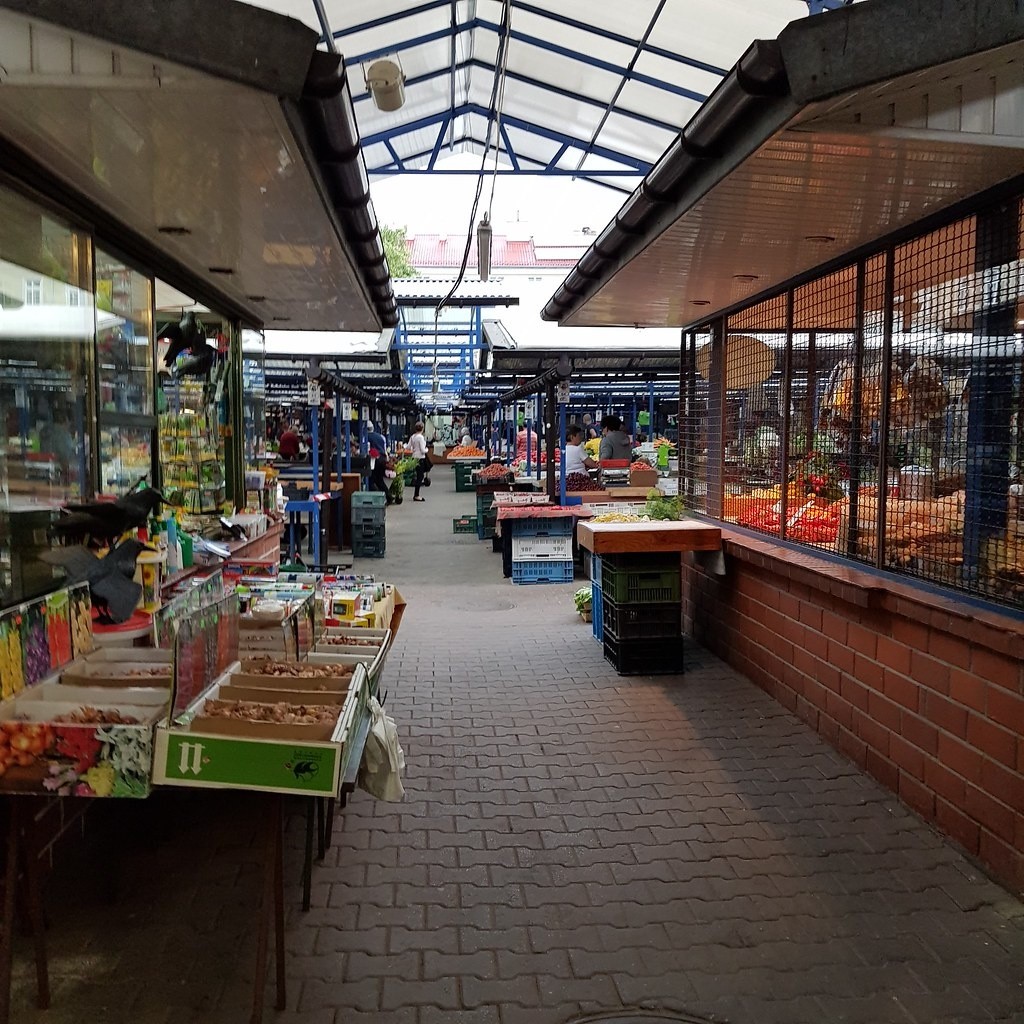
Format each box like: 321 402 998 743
419 453 433 473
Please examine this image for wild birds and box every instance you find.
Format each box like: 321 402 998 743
157 310 217 381
37 487 174 625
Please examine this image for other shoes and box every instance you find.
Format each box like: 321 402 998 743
413 496 425 501
385 497 394 506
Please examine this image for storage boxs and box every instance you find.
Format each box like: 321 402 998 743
602 552 683 604
2 386 408 803
603 629 684 676
446 415 714 587
603 597 682 641
591 583 603 645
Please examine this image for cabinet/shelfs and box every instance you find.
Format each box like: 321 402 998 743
1 185 95 515
89 242 153 497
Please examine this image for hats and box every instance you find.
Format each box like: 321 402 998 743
362 420 373 428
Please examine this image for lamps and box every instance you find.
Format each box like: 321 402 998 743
431 362 440 396
477 219 493 284
361 48 407 113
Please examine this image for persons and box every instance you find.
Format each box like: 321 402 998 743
599 415 632 460
408 422 429 501
278 426 300 460
583 414 598 441
367 420 395 505
517 420 537 452
566 424 596 477
457 427 472 447
752 408 863 447
